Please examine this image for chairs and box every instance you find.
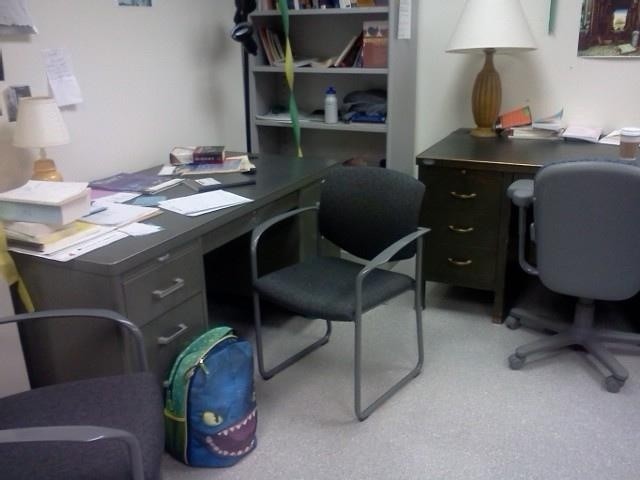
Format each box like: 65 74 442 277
250 165 434 422
504 160 639 393
0 308 165 480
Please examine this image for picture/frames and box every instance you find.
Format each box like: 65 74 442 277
577 0 640 59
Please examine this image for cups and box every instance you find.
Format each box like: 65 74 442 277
619 127 640 161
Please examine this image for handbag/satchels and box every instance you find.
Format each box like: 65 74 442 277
159 326 257 469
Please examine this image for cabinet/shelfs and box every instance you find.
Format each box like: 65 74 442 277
242 0 418 178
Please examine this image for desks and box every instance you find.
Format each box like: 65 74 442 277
3 151 338 397
415 128 639 326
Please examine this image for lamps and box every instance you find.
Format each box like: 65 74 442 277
10 97 70 183
447 0 539 140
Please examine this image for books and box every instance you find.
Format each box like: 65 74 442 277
508 107 565 141
0 219 100 252
193 146 225 164
562 127 602 143
274 56 319 68
87 172 185 195
182 171 256 193
334 30 363 67
259 24 286 66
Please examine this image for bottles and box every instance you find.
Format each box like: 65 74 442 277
324 87 339 124
